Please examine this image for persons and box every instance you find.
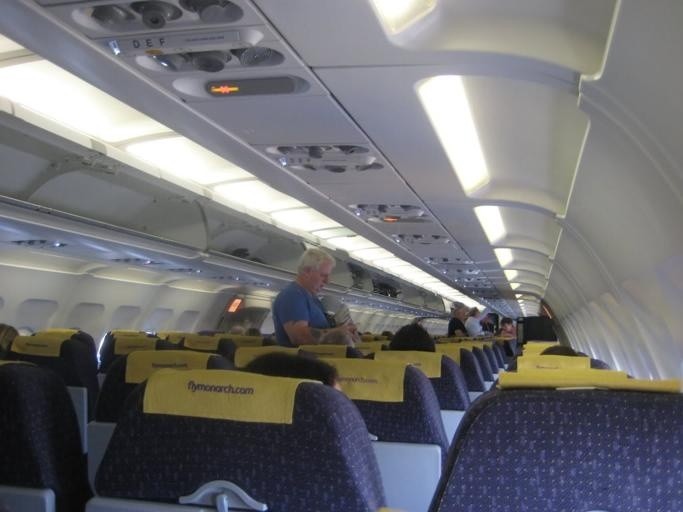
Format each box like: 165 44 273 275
464 308 493 336
272 248 357 347
448 302 467 336
500 318 515 337
